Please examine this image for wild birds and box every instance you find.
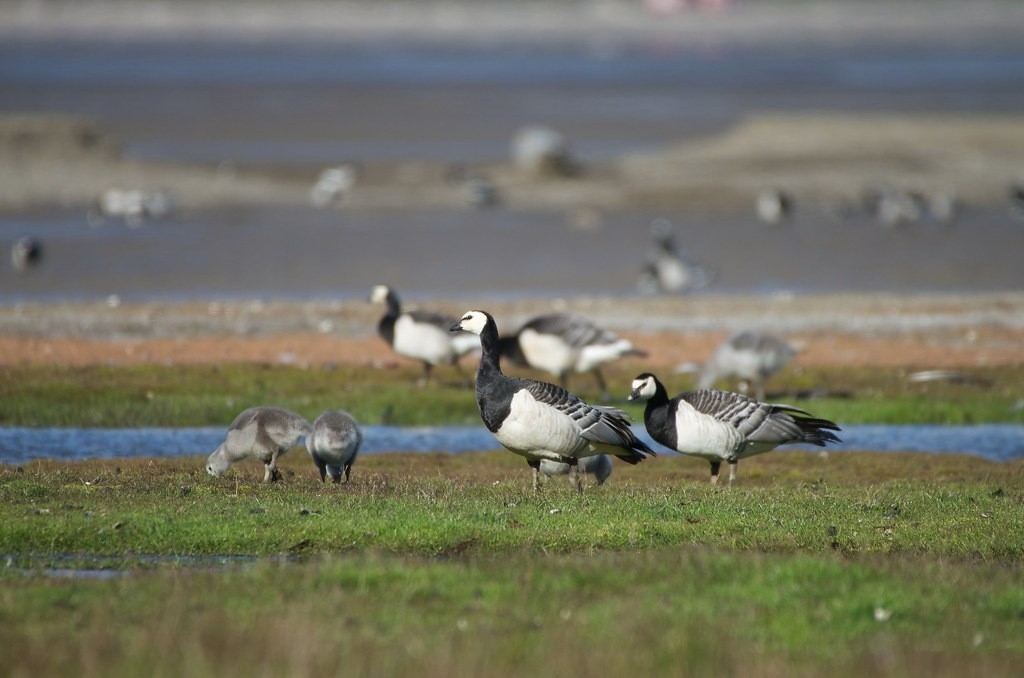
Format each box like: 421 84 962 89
628 372 844 488
449 310 657 498
541 454 613 486
699 330 812 402
500 313 649 402
205 408 310 484
366 285 500 389
305 409 362 484
